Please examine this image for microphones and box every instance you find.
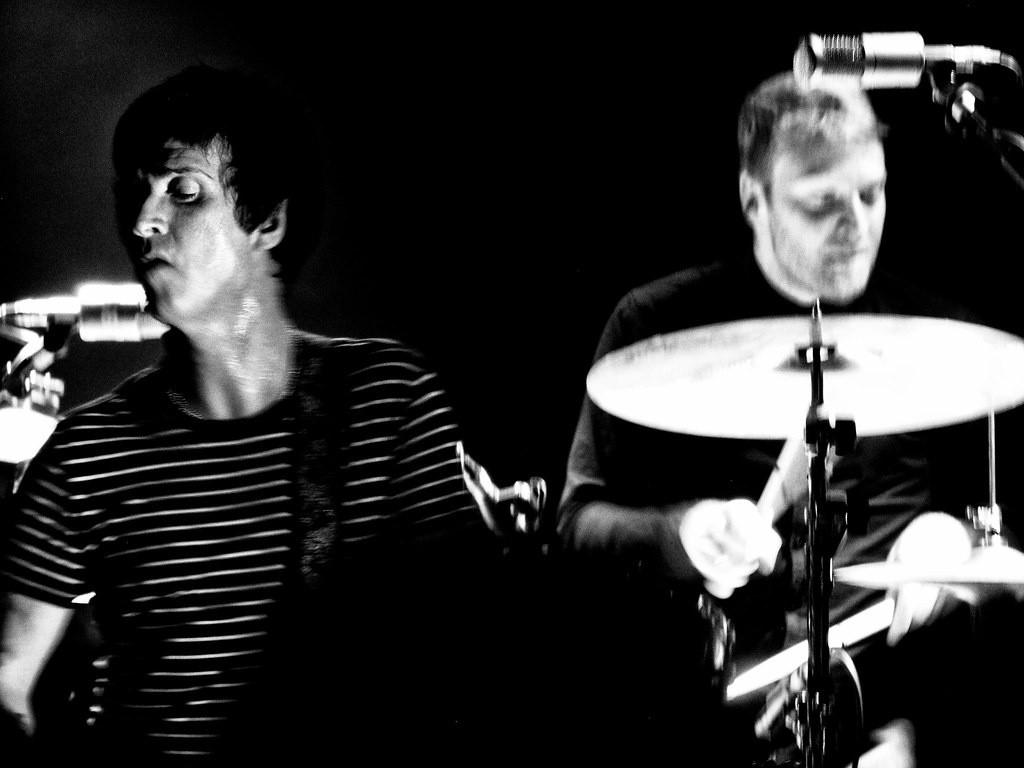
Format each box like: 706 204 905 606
795 32 1002 89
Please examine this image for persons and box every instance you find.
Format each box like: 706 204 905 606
220 504 753 768
554 68 1024 768
0 61 483 768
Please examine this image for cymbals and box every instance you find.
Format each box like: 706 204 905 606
586 312 1024 443
836 544 1024 595
